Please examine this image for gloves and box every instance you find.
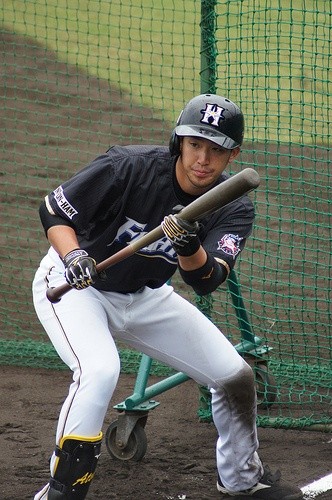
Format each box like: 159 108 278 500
161 204 201 256
63 249 107 290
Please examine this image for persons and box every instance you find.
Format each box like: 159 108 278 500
31 94 302 500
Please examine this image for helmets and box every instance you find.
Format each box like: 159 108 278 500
169 94 244 158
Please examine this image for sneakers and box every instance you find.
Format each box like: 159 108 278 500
217 475 303 499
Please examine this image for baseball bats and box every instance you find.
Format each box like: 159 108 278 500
45 167 261 304
